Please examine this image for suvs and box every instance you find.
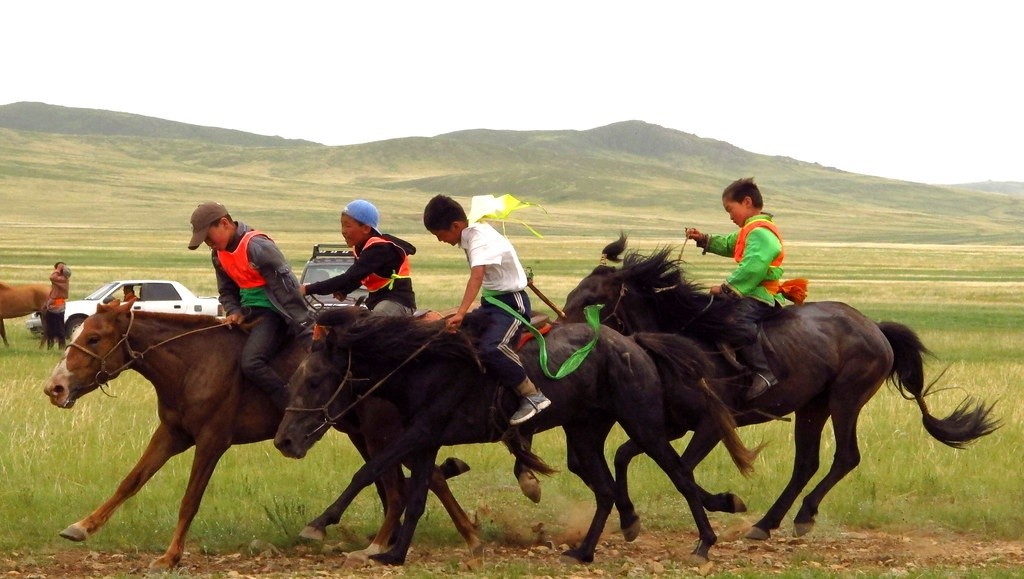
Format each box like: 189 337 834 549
301 244 370 312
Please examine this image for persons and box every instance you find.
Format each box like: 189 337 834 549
686 177 785 400
123 285 138 301
299 200 417 319
190 201 310 414
45 262 69 351
423 194 551 425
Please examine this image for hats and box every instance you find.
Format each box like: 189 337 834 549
188 202 228 250
342 200 382 235
63 266 72 278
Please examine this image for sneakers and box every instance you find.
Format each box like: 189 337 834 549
511 388 551 424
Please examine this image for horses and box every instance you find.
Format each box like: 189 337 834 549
0 282 63 349
270 289 770 564
553 226 1007 544
35 294 510 567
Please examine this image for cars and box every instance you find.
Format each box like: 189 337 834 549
27 279 220 343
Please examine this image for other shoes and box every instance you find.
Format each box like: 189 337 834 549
747 370 778 400
